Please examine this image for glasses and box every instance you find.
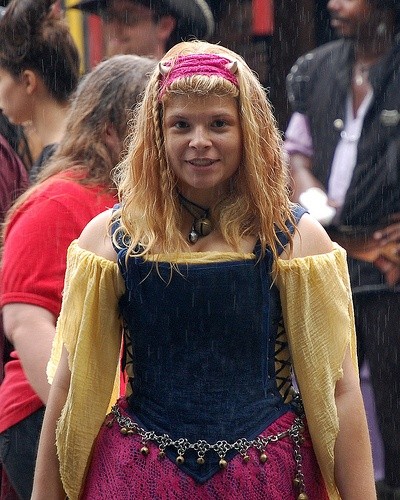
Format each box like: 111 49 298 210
101 5 163 26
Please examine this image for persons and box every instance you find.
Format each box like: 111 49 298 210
0 53 156 500
283 0 400 500
30 40 378 500
0 0 79 227
214 0 317 143
70 0 213 62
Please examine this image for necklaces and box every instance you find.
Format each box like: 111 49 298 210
175 188 231 245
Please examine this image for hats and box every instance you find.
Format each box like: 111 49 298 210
74 2 217 41
